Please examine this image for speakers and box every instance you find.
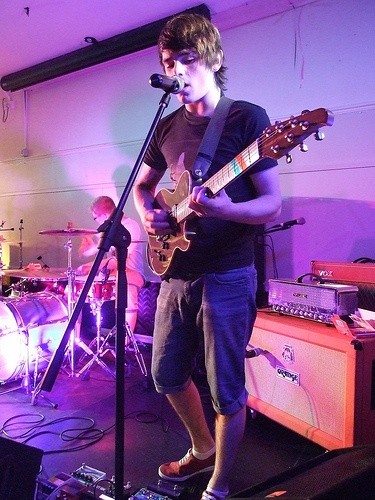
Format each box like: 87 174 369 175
243 307 375 451
0 437 44 500
227 444 375 500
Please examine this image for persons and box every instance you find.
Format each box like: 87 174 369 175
77 196 146 346
131 12 283 500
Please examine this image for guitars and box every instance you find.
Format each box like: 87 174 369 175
145 107 335 280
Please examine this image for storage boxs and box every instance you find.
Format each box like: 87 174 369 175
244 308 375 451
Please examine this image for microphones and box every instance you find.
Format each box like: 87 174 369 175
273 216 305 228
244 347 262 359
148 73 185 94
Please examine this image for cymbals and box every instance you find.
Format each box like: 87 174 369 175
40 228 99 237
4 265 64 282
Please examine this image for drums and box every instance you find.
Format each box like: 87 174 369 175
23 273 68 294
74 274 115 305
0 291 75 384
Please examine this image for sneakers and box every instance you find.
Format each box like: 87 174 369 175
158 448 216 481
201 491 230 500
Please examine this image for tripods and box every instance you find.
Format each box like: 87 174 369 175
0 233 152 408
